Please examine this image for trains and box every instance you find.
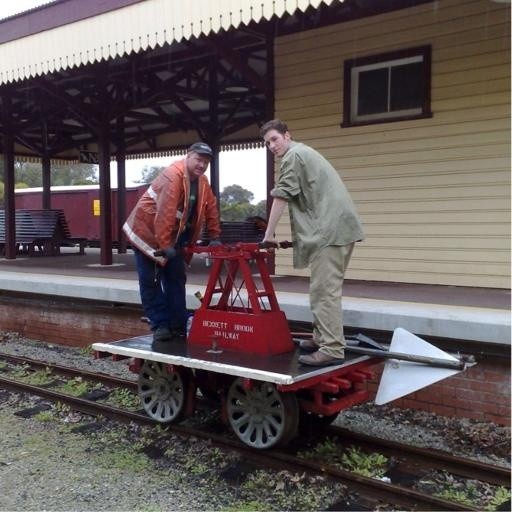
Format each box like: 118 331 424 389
13 181 154 250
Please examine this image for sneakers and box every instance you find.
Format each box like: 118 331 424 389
153 326 186 340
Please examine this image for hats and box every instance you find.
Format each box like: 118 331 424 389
190 142 215 159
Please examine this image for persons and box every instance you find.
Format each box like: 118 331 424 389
259 119 364 365
123 142 222 342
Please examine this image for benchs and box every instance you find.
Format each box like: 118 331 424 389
0 207 89 257
199 221 268 247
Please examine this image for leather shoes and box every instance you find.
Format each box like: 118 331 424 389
298 339 345 365
